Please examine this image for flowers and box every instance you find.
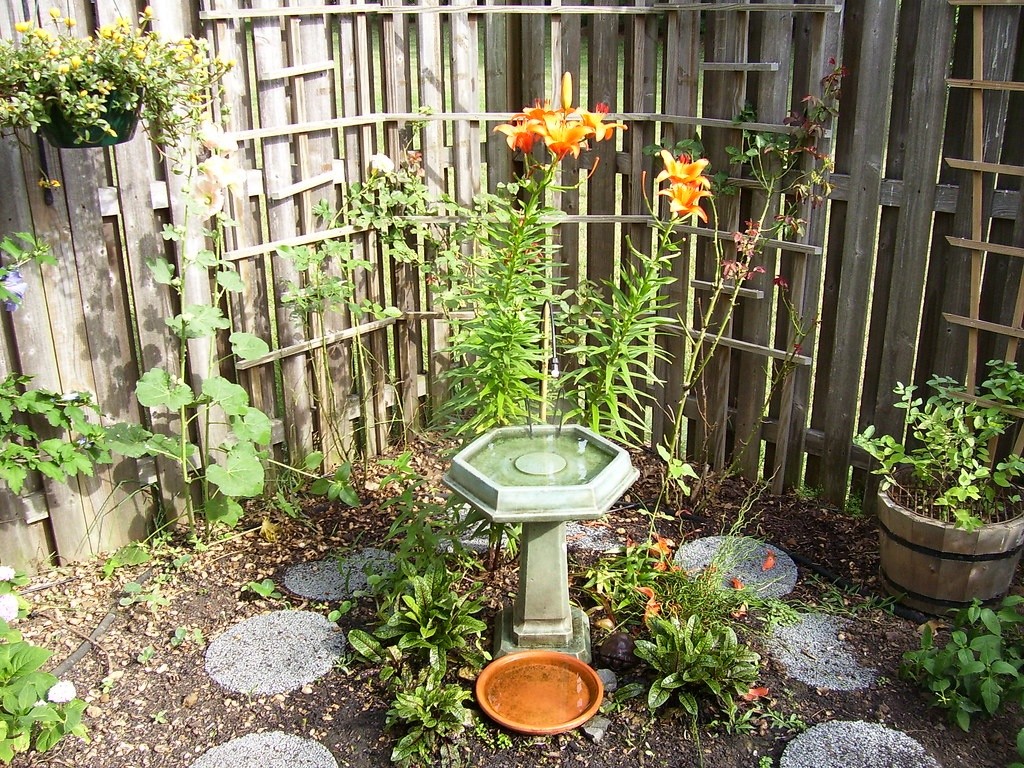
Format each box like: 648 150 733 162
0 4 239 208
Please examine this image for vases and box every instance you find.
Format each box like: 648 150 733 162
38 80 144 148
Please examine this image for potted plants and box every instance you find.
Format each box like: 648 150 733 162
846 358 1024 622
